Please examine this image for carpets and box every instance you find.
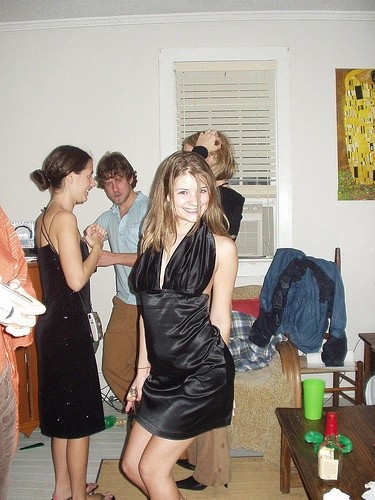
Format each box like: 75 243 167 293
93 456 309 500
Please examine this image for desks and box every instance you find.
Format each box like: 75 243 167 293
275 404 375 500
358 332 375 382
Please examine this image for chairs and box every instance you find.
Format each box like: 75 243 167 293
300 247 363 407
229 284 302 459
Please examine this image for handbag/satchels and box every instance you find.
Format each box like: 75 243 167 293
88 310 103 342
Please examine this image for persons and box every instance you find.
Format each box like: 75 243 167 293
121 150 239 500
29 144 116 500
0 204 46 500
95 129 245 491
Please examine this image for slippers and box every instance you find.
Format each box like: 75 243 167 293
50 483 116 500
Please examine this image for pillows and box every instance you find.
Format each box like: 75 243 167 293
231 297 260 318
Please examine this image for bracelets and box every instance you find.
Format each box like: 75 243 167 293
137 367 151 369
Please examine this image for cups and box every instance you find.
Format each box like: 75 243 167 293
303 379 325 420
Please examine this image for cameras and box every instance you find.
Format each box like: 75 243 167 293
87 312 103 342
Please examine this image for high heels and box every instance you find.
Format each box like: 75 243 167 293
176 459 196 471
176 475 229 491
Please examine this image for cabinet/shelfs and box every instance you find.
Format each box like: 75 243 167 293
14 262 42 439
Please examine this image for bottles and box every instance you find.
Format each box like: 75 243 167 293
318 412 343 485
103 415 117 429
